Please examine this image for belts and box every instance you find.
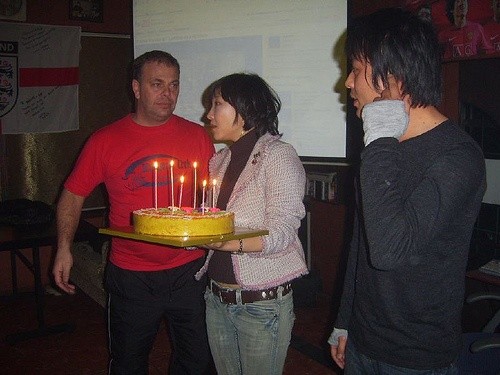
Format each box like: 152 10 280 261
206 282 291 305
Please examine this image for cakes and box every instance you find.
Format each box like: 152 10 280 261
131 206 235 237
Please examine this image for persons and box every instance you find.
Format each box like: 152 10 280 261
195 74 309 375
52 50 216 375
328 7 487 375
415 0 500 60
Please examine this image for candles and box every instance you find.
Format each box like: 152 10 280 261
154 160 215 215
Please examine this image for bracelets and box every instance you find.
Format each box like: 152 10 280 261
238 239 243 252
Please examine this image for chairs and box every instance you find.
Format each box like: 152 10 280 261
68 240 109 308
460 291 500 375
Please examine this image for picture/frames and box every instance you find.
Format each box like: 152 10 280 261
69 0 104 22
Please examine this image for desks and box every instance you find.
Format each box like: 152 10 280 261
0 199 98 344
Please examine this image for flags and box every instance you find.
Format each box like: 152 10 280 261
0 22 81 134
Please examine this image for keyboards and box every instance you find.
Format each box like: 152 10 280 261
480 260 500 277
